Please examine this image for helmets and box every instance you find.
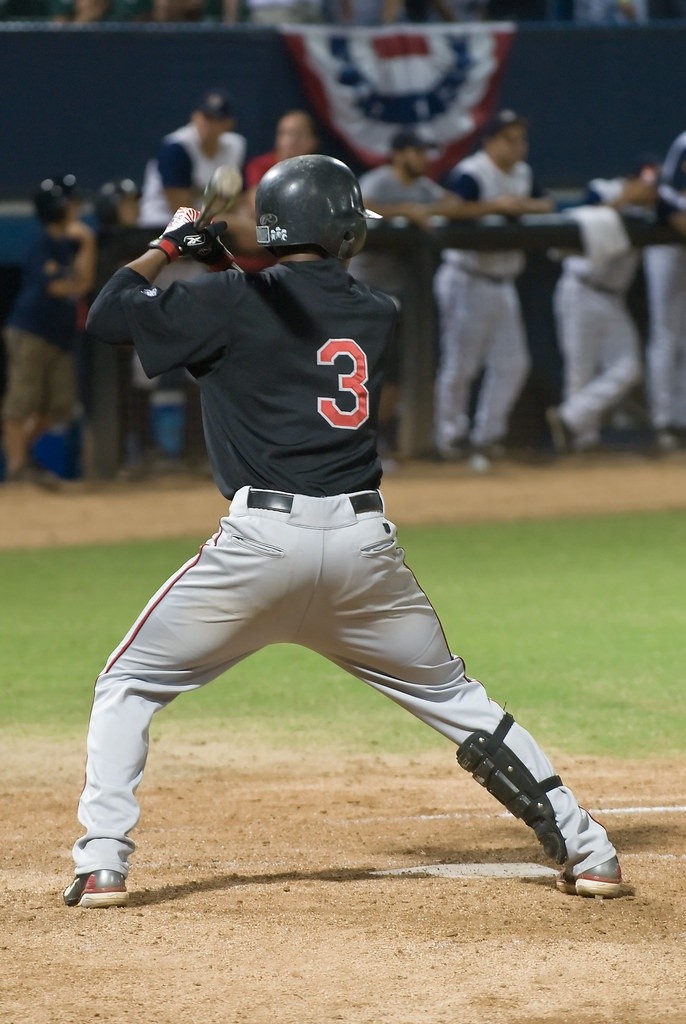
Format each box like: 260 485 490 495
254 152 384 259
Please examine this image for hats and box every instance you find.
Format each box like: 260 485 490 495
480 109 527 145
195 88 234 122
391 126 442 150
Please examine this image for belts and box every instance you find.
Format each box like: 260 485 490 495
246 488 384 515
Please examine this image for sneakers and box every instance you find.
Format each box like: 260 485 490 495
555 855 621 897
62 869 129 907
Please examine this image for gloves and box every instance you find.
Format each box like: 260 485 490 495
148 206 234 274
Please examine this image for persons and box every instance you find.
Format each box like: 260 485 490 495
63 154 625 906
0 0 686 488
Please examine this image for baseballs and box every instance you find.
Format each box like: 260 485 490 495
193 165 243 228
